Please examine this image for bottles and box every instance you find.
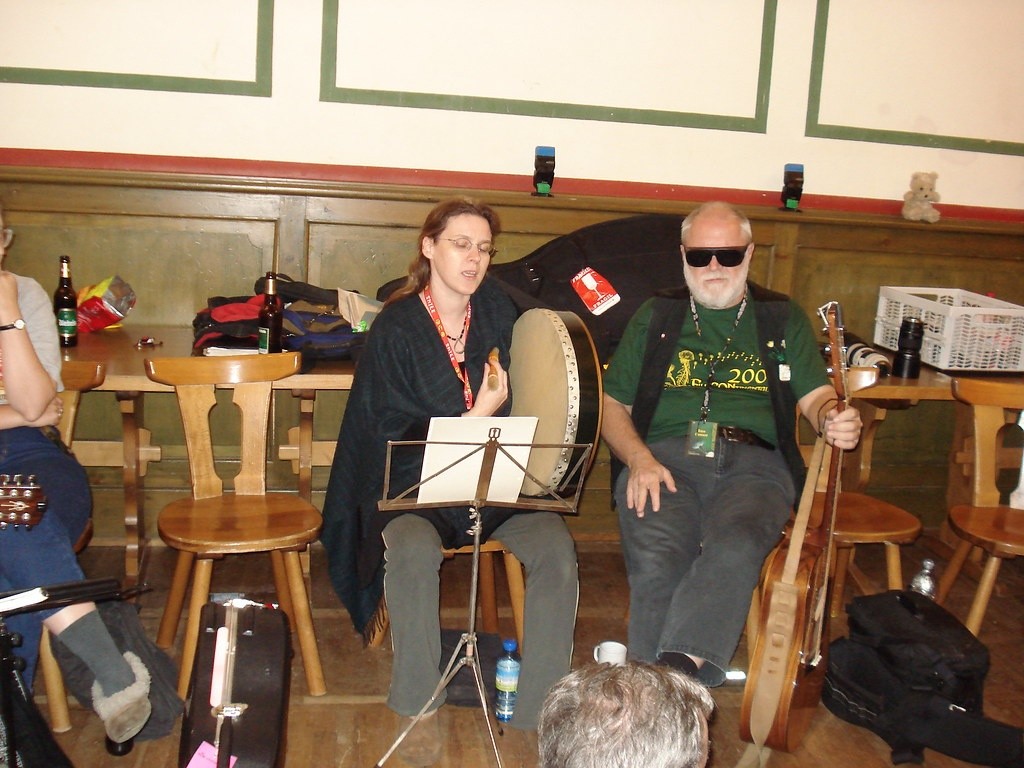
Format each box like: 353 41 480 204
258 272 282 356
53 255 78 346
494 641 519 721
911 559 939 603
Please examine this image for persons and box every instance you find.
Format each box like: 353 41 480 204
1 215 149 756
600 201 864 691
319 200 580 768
537 663 715 768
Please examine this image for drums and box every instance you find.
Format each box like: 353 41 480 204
506 307 604 498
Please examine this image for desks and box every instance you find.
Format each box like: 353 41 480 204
60 324 362 612
793 345 1024 565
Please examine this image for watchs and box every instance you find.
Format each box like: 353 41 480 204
0 319 27 333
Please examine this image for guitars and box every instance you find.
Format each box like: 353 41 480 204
0 471 50 533
736 299 853 753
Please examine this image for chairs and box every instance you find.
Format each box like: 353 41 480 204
746 380 1024 673
39 363 106 733
144 350 326 702
368 536 525 658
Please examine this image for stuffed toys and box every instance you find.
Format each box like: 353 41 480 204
902 172 942 223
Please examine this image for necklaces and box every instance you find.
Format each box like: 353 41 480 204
444 313 469 355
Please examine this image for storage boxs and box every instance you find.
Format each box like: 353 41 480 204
873 284 1024 373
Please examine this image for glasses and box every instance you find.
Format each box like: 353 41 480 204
434 238 498 256
682 242 751 267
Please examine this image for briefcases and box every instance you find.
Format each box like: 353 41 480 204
178 602 294 768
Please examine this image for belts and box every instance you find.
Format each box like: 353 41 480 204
716 426 776 452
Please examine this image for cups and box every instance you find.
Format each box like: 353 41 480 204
594 642 627 666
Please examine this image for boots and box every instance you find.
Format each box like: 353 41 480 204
91 652 150 755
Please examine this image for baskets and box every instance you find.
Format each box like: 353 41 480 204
874 286 1024 372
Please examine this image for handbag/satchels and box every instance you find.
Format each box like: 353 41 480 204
190 272 369 361
824 587 1023 768
